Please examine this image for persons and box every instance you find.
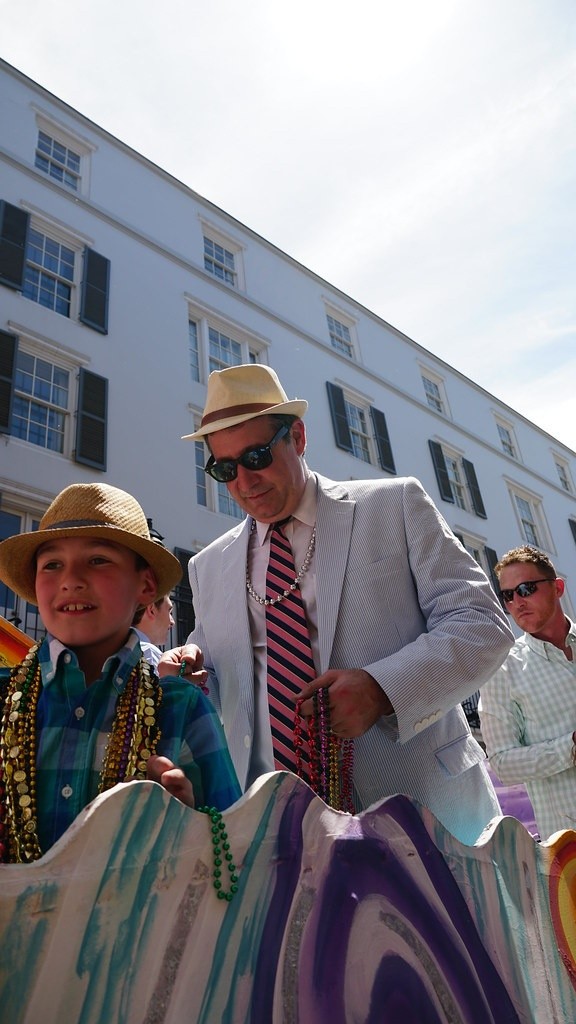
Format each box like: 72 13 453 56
132 591 177 681
158 363 517 846
0 481 244 862
477 544 576 841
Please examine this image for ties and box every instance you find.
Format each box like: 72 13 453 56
265 517 322 803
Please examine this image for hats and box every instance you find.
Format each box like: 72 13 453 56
180 364 308 441
0 482 183 612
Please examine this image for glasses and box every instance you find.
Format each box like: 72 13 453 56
497 578 554 602
203 425 291 482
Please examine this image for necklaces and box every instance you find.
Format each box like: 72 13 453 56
243 516 318 605
293 687 355 817
1 635 164 866
196 804 240 903
